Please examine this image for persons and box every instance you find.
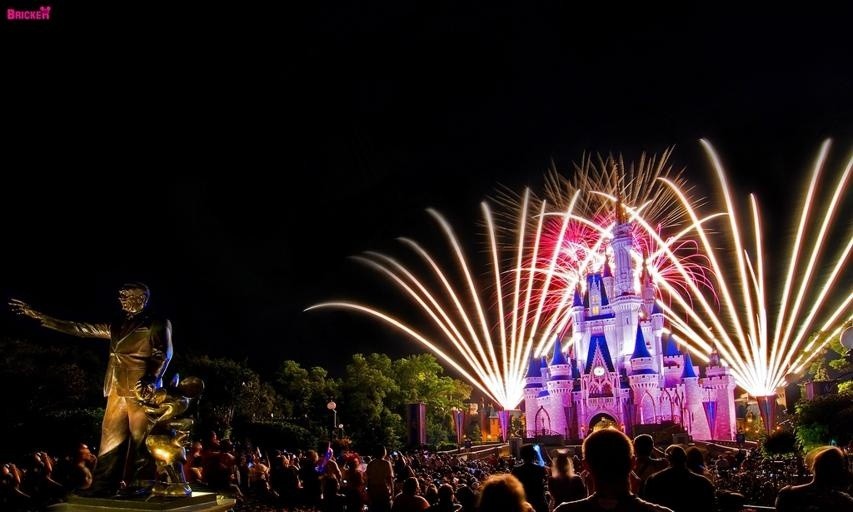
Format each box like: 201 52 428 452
6 280 174 497
